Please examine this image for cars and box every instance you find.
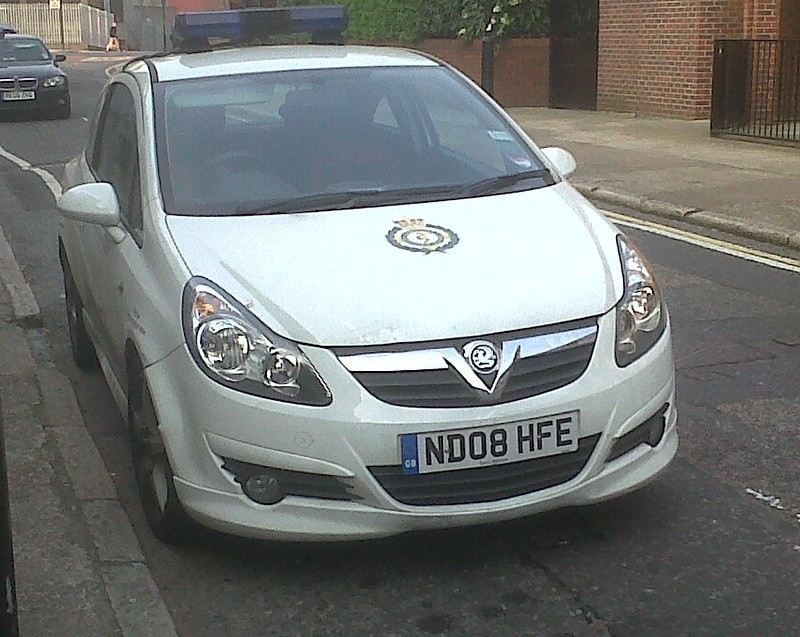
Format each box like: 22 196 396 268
52 42 680 545
0 33 72 119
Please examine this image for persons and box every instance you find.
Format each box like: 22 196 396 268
106 23 121 52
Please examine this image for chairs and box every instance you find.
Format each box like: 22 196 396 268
163 106 225 135
285 90 333 125
